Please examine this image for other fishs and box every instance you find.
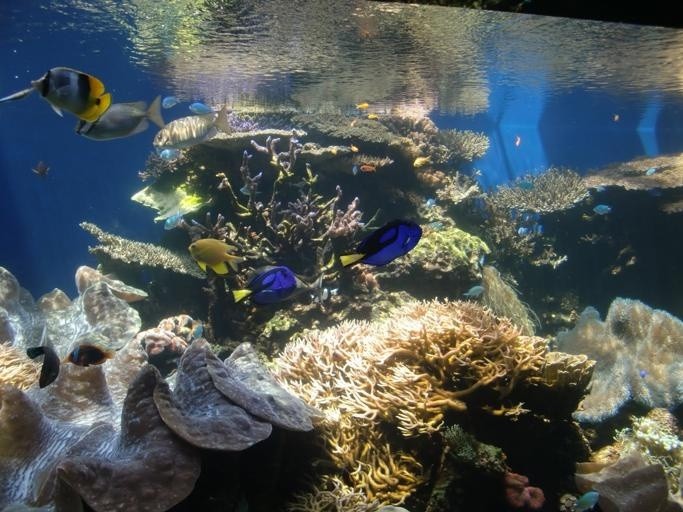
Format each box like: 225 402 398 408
1 66 683 310
